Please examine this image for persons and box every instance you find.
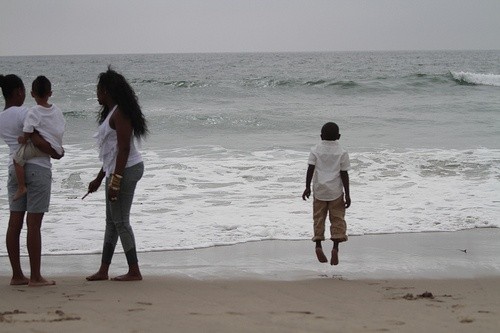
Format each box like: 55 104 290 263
12 76 66 200
0 74 65 286
86 70 144 281
302 121 352 265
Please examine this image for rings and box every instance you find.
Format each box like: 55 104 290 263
112 197 117 201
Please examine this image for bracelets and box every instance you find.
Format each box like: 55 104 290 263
109 174 123 190
